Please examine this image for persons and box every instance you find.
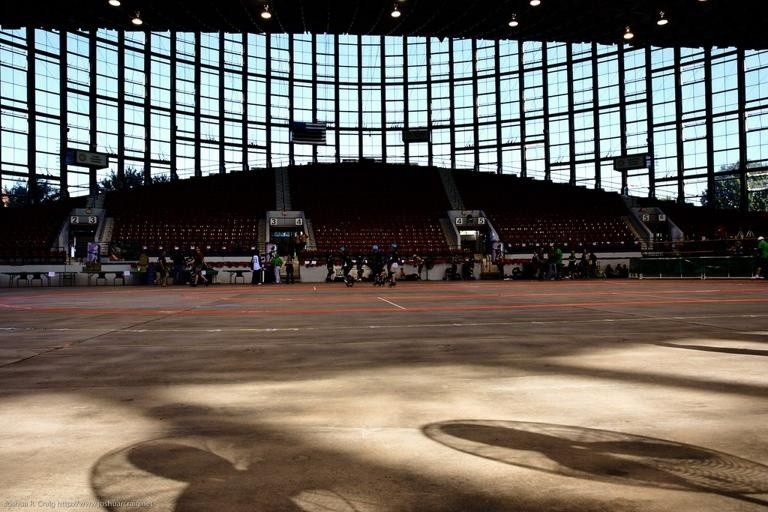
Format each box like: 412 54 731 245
675 229 768 279
149 243 208 286
251 249 296 285
326 245 424 287
532 243 629 280
